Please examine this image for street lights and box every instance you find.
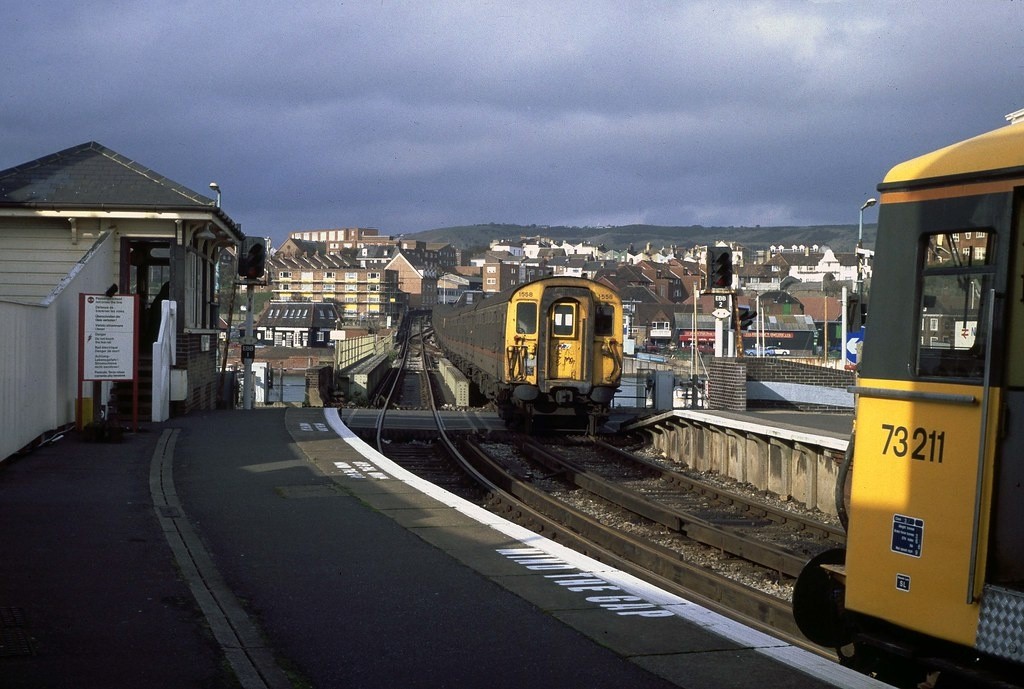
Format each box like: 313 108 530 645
209 183 222 303
859 198 877 297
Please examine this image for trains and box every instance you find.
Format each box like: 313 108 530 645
791 107 1024 689
431 276 624 431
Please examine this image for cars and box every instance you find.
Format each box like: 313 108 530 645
767 346 791 356
745 347 774 357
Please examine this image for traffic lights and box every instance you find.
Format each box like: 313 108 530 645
238 236 264 278
707 247 732 288
